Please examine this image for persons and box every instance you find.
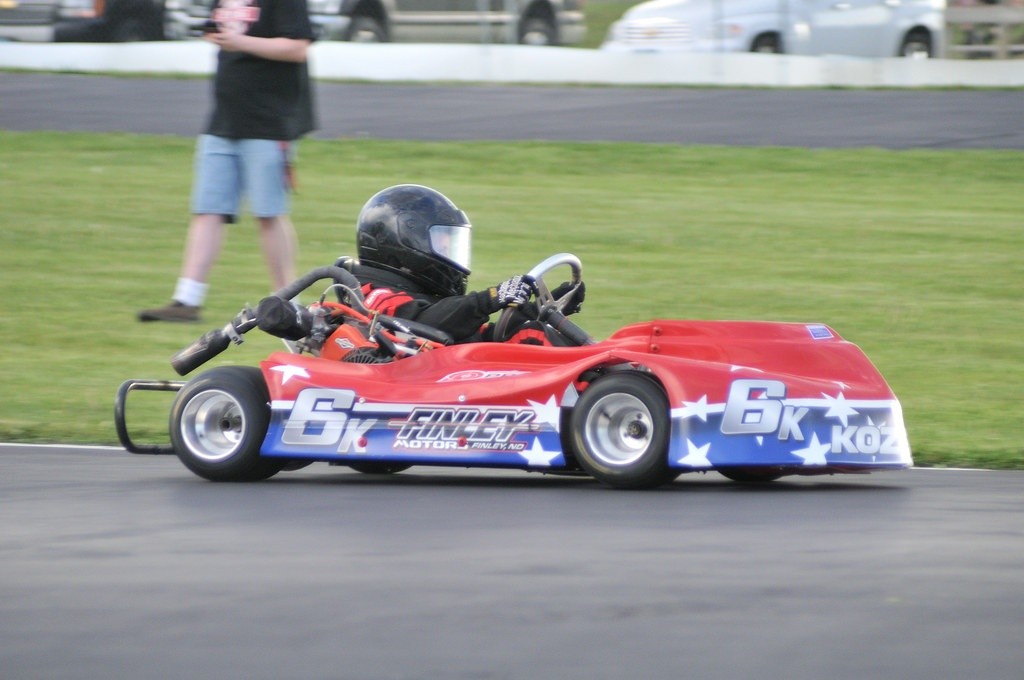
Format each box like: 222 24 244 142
137 0 318 321
332 184 586 349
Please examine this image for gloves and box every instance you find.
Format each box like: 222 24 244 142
488 275 540 312
551 281 585 316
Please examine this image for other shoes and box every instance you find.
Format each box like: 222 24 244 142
141 301 202 324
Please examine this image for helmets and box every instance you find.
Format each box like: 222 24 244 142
356 184 472 298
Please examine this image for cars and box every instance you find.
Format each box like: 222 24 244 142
0 0 589 44
602 1 949 58
